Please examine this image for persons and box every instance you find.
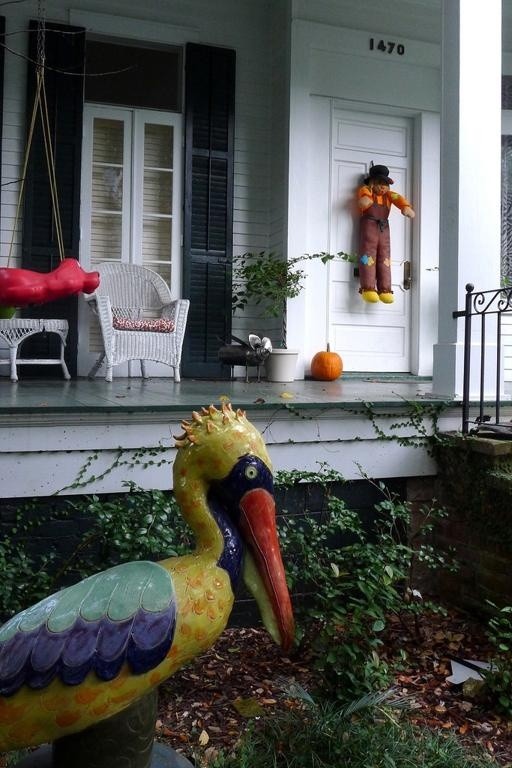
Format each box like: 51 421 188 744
356 162 417 306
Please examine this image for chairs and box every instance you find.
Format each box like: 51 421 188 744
80 262 190 384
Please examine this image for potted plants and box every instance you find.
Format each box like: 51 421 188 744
226 250 358 382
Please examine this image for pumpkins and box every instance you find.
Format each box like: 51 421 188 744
310 343 343 381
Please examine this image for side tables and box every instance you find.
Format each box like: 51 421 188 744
0 317 70 384
